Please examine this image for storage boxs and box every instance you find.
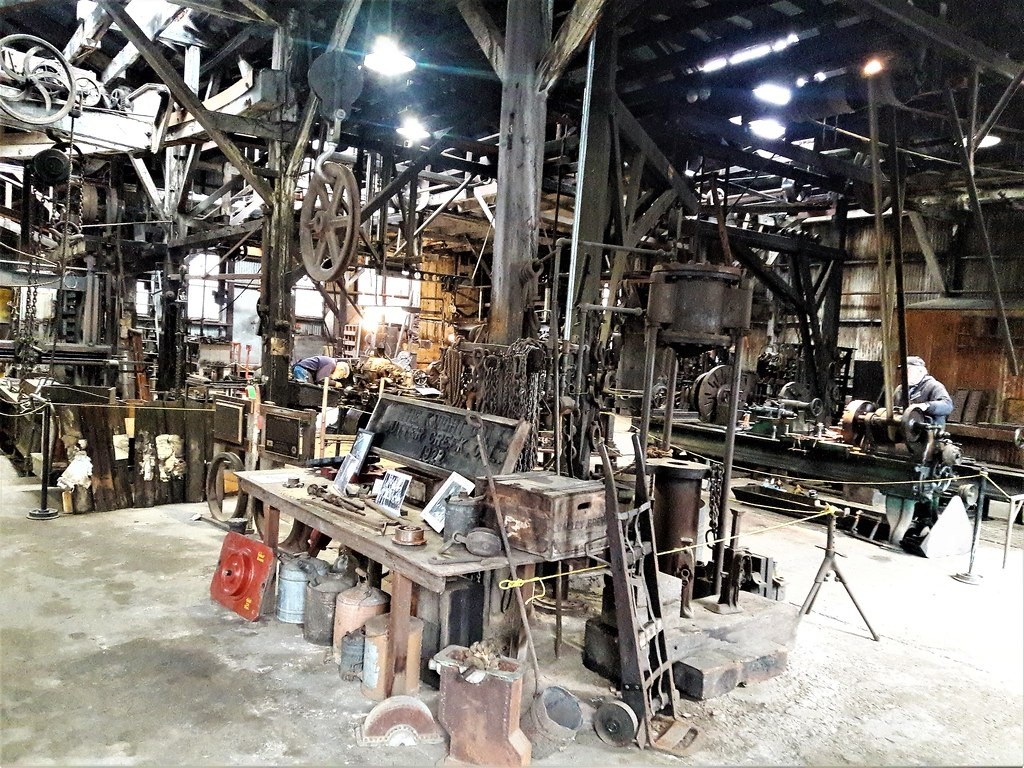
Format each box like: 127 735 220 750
475 470 608 560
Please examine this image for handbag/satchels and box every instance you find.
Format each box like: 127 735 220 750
290 358 302 374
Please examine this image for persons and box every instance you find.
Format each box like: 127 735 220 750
892 356 953 445
293 356 350 388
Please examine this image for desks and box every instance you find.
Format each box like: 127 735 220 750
236 467 546 697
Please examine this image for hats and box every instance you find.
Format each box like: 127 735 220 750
897 355 925 370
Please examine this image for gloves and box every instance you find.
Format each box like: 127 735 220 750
912 402 930 411
